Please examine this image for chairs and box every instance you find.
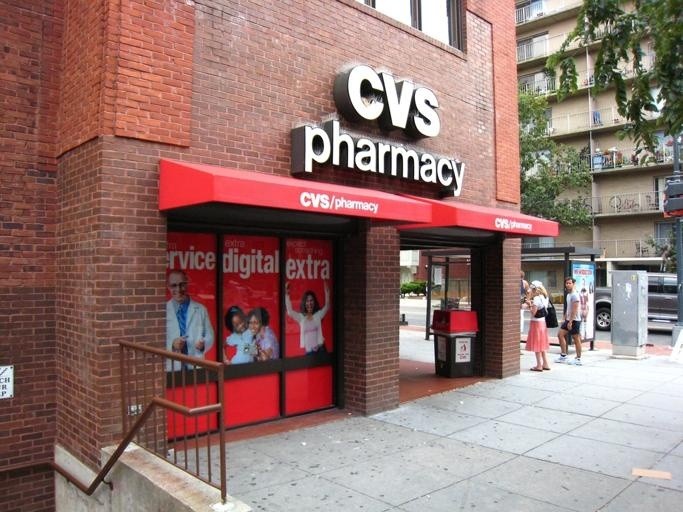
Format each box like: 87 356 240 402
635 240 649 257
646 196 659 209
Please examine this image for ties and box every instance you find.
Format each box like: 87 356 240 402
176 305 188 354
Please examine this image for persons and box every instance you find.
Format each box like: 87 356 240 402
166 268 214 372
555 277 582 366
524 280 550 371
520 271 531 334
223 305 280 365
285 281 330 356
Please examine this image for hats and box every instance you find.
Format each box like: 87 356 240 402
530 281 542 289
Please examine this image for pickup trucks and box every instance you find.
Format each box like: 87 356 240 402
595 273 678 331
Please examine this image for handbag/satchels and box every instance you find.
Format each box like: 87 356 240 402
546 306 558 328
535 307 547 318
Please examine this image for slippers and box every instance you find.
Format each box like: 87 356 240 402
530 366 550 371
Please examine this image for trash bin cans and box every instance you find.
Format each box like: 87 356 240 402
431 310 479 378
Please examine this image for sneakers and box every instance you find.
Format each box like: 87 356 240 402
556 355 581 365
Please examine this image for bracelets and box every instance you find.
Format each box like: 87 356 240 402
529 306 533 308
